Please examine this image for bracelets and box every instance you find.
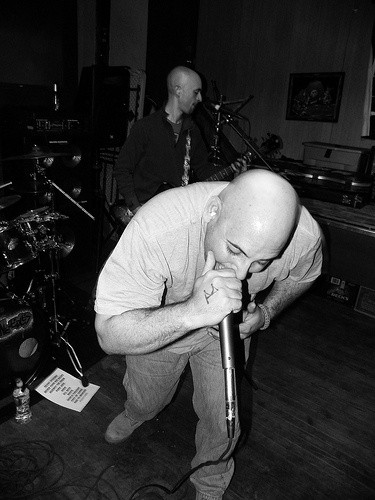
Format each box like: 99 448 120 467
258 303 270 331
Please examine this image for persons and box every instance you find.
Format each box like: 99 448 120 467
113 67 247 215
94 169 325 500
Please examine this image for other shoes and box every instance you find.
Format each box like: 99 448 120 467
196 490 222 500
103 410 144 445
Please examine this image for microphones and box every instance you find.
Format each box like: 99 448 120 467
205 99 244 119
217 313 236 439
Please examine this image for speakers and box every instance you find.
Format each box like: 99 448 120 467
81 66 130 149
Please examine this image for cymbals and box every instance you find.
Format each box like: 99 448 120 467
0 195 21 212
35 118 80 131
6 153 82 161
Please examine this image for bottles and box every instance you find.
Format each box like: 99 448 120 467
13 379 33 425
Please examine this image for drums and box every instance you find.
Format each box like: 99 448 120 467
14 207 55 259
0 222 41 273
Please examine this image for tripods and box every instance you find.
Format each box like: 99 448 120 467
0 172 88 386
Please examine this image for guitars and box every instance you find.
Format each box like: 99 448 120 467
118 131 285 225
